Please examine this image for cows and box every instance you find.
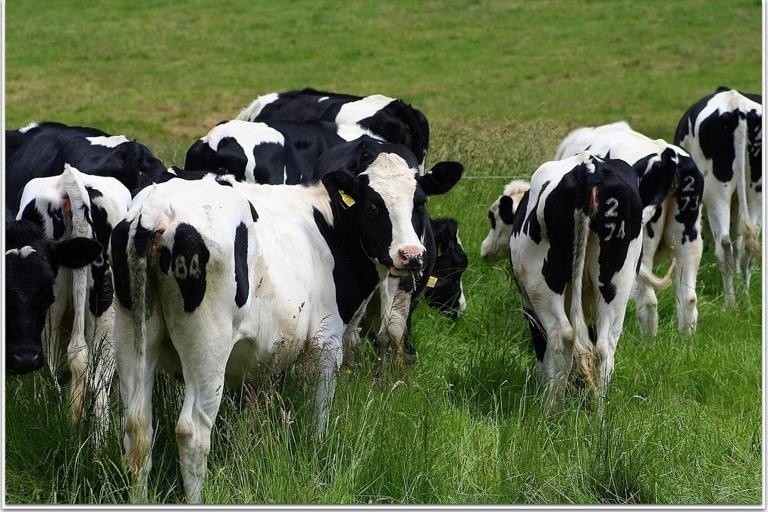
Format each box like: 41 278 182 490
111 154 467 506
5 87 470 470
479 150 644 437
673 85 762 314
555 120 704 345
